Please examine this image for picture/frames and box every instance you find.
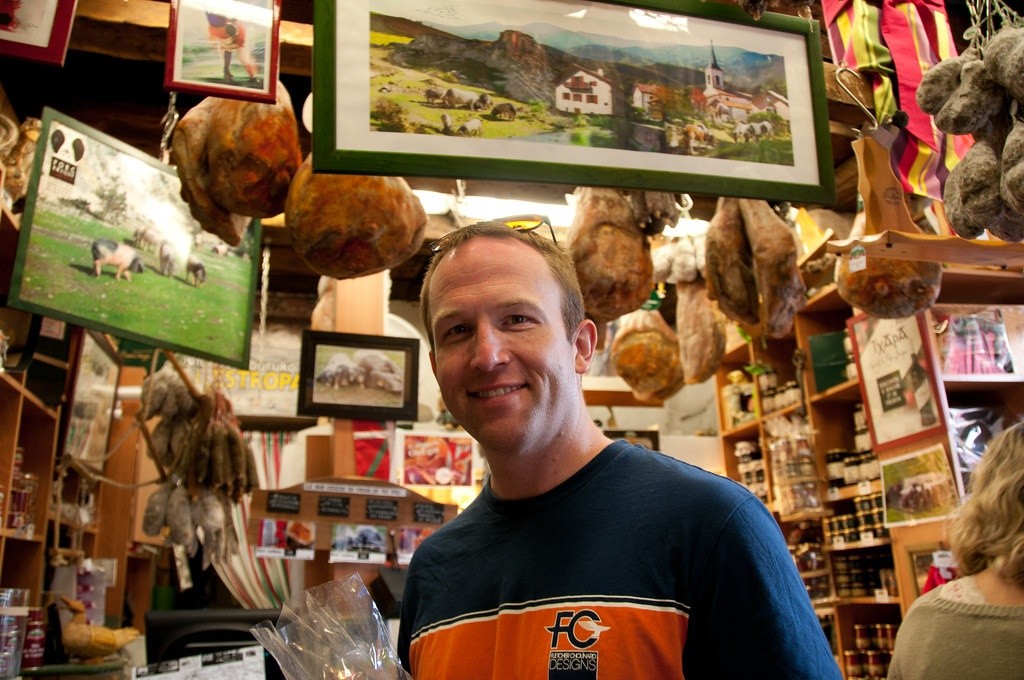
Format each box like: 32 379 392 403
164 0 282 105
6 106 263 374
294 329 421 422
604 430 660 453
901 541 960 598
311 0 838 209
61 327 123 474
0 0 78 67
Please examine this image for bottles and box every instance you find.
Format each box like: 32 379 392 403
765 434 817 516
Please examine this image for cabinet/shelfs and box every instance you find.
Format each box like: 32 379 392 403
0 305 169 680
712 266 1024 680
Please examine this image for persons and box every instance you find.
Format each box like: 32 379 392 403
205 11 261 85
398 224 843 678
886 420 1023 679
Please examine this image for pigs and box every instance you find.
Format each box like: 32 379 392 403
89 218 226 290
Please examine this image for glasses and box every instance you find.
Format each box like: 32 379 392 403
429 214 557 256
